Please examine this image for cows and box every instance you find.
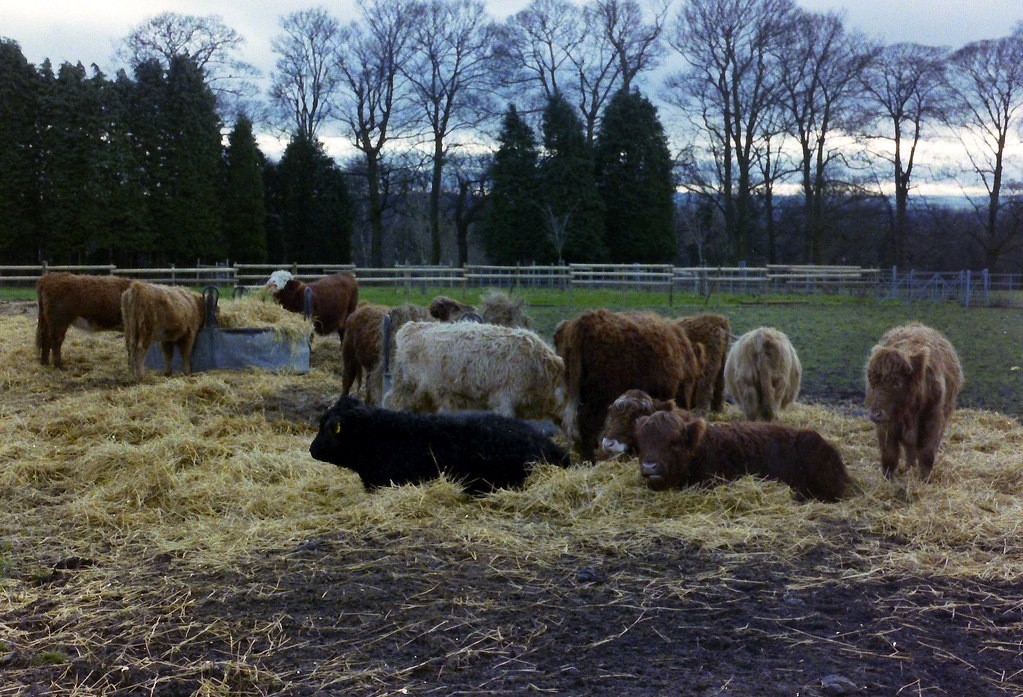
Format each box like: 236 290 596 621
265 269 358 355
864 322 963 484
309 293 852 503
34 271 222 382
